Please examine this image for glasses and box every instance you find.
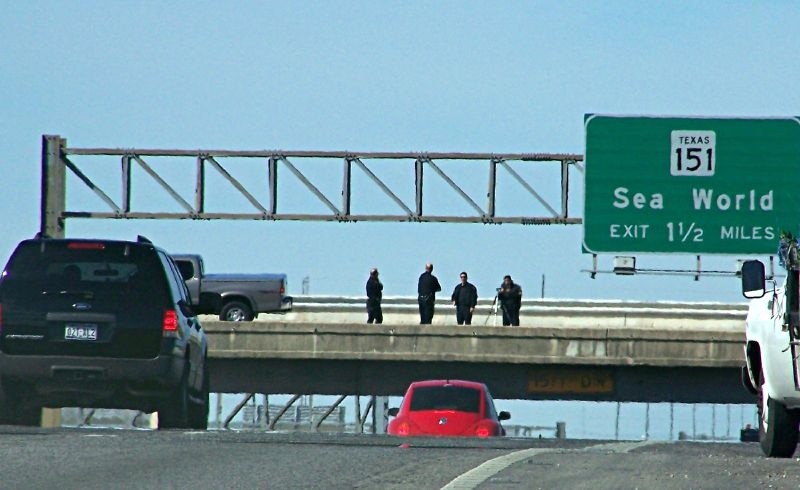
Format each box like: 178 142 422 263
461 275 467 279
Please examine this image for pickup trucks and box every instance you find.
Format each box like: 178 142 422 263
170 253 293 321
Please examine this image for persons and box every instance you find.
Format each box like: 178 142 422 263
497 275 523 327
451 271 479 325
417 262 441 325
366 267 383 324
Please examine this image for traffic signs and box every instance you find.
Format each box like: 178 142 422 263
581 112 800 257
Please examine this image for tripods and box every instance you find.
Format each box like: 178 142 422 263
485 295 512 326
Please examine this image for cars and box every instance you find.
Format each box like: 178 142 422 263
386 379 511 438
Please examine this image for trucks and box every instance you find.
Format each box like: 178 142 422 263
741 258 800 458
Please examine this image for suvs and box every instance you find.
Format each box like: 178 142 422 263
0 234 222 430
